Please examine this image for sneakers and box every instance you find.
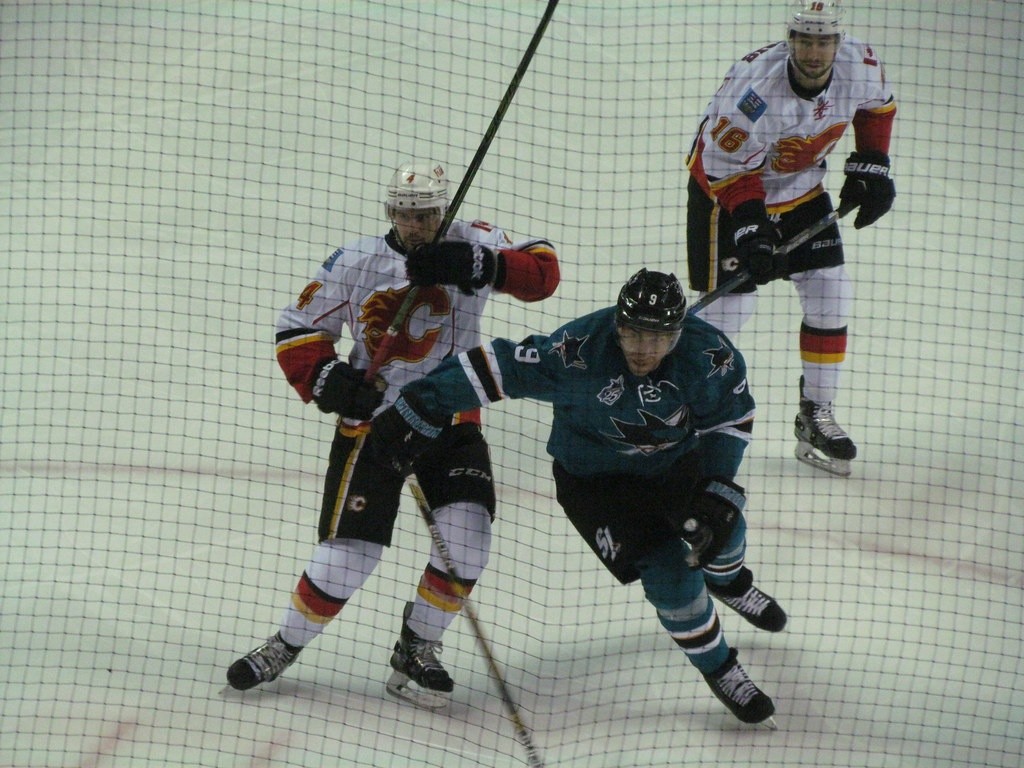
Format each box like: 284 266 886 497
794 373 856 477
217 631 304 696
706 566 787 633
703 648 778 730
386 603 454 708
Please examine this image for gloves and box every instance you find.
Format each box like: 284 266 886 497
311 361 388 421
417 241 495 289
731 199 788 285
369 391 446 469
837 149 896 229
676 475 745 571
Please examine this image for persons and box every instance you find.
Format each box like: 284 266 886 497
685 0 898 481
370 267 788 730
220 159 562 709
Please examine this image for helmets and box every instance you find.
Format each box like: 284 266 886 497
384 161 450 226
615 268 688 335
787 0 846 48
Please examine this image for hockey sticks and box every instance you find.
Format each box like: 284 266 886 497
682 198 860 322
392 455 543 768
358 0 557 389
